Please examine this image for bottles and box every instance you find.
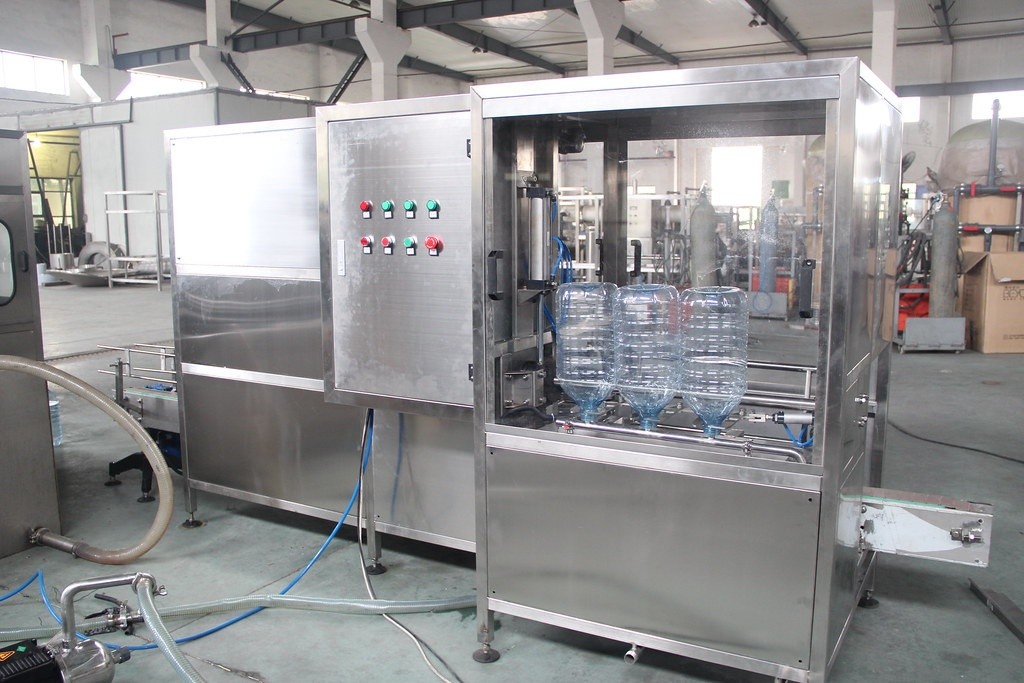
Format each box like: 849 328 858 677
553 283 619 422
612 284 681 430
48 385 65 446
680 286 750 438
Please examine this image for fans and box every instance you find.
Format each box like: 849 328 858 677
903 150 916 173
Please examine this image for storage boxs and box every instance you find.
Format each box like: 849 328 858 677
958 251 1024 354
